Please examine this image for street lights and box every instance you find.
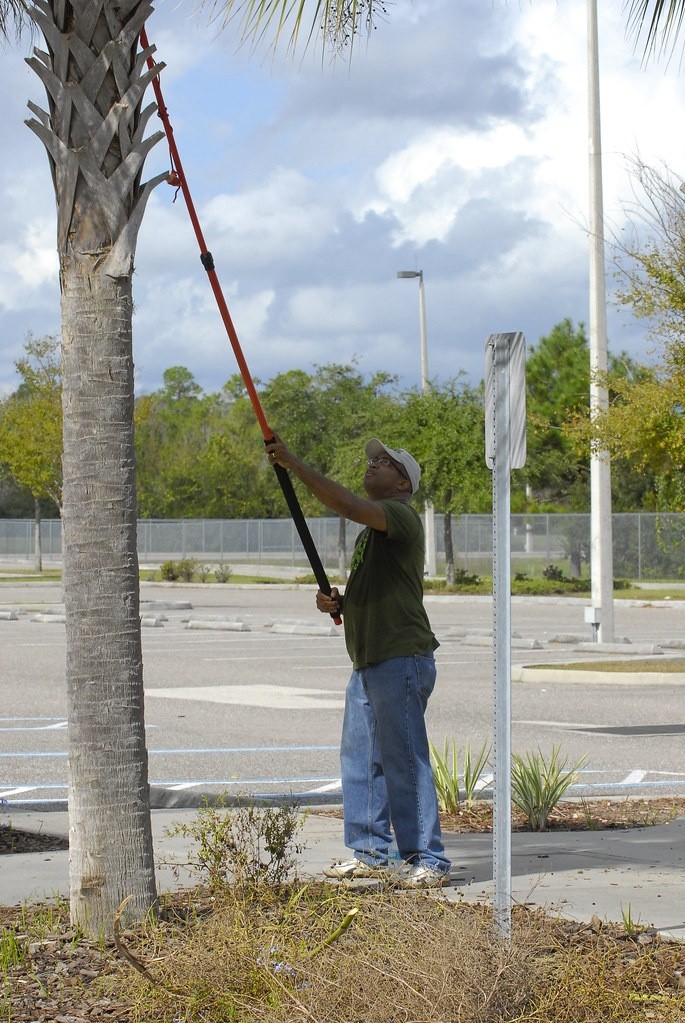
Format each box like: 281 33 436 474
394 264 439 579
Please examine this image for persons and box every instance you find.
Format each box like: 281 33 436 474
264 426 452 889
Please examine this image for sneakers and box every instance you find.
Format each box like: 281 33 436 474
388 865 451 888
323 857 390 878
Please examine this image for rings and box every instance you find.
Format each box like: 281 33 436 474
272 453 275 458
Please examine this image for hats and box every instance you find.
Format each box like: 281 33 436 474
364 438 421 496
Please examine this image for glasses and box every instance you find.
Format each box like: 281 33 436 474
367 458 408 481
